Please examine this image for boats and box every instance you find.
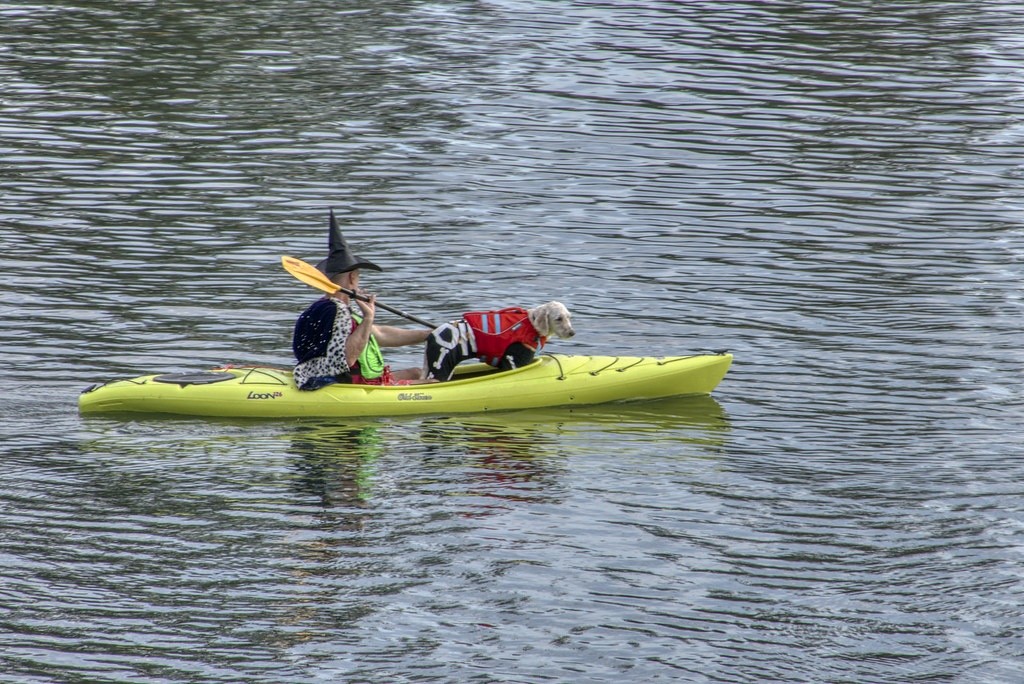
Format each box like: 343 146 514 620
79 350 734 420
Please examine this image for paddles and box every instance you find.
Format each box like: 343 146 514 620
279 252 439 331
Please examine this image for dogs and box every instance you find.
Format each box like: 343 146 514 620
418 301 576 382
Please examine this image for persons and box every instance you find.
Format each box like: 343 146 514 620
292 246 440 387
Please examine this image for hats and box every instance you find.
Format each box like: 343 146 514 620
306 208 383 288
